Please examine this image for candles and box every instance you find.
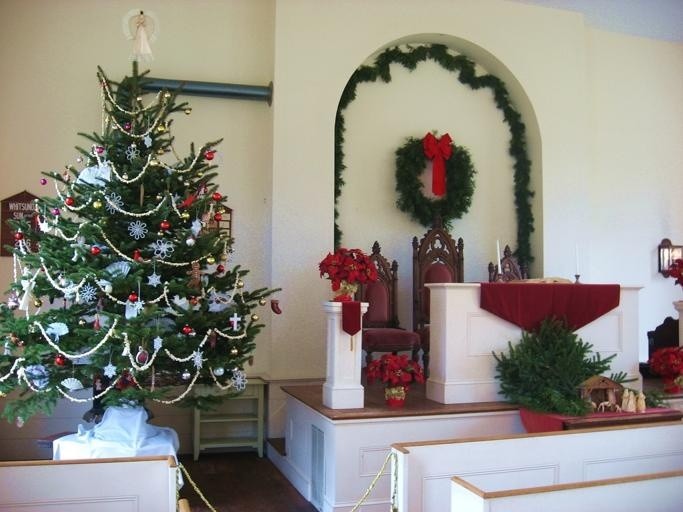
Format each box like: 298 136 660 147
496 238 500 274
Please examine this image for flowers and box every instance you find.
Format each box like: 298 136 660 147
318 248 379 292
648 345 683 388
662 253 682 290
365 351 426 399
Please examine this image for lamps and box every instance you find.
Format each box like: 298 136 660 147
658 237 682 279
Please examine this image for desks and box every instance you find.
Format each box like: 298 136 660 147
425 280 644 407
41 426 175 461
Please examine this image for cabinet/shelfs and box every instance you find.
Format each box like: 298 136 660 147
191 377 267 462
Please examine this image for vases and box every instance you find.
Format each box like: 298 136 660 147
335 284 356 302
663 376 679 394
383 385 406 408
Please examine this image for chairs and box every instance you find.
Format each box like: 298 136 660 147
357 239 421 384
486 244 527 283
412 225 466 380
